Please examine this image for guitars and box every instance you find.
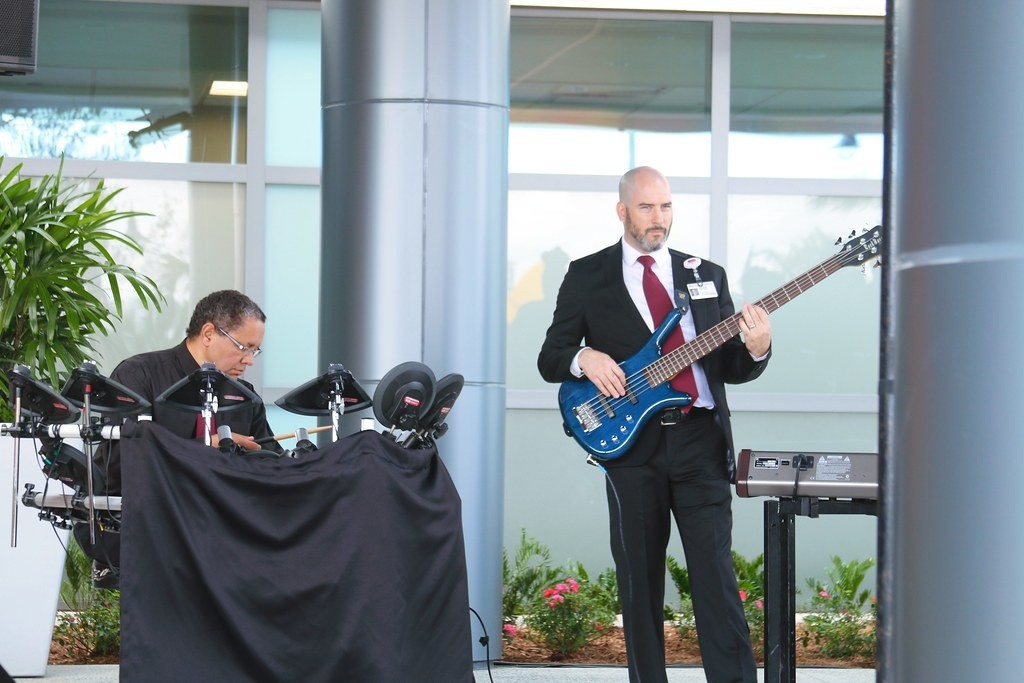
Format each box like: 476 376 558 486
558 224 882 461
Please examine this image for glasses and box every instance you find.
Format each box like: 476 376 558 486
213 323 262 358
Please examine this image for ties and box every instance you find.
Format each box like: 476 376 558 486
196 410 215 440
637 256 698 415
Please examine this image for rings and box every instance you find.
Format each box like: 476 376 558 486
749 325 755 329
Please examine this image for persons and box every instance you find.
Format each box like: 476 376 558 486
537 167 773 682
71 289 286 567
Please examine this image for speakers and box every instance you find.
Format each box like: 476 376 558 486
0 0 41 72
